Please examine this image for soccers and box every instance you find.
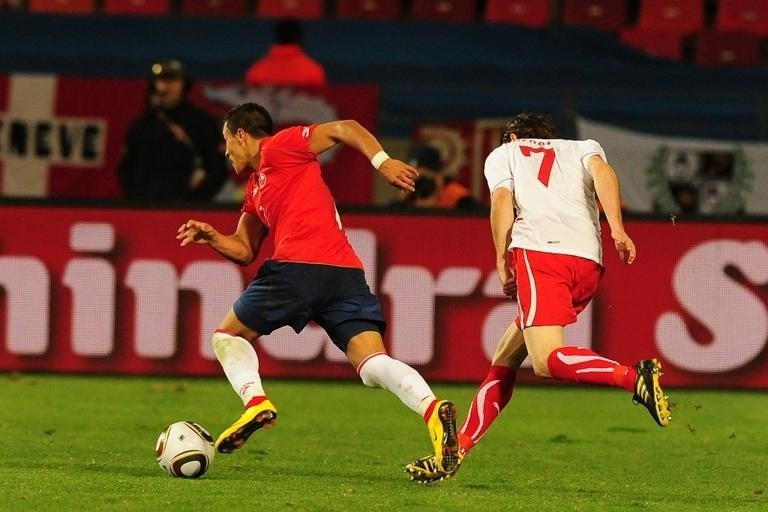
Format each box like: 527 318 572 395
155 419 215 480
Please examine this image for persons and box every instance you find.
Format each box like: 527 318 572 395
401 110 674 487
389 145 486 211
238 14 328 89
175 100 462 481
113 53 232 206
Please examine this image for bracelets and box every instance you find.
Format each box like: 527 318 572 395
370 148 389 169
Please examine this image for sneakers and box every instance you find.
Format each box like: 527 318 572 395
427 399 460 475
215 396 278 454
633 359 672 427
404 452 462 485
